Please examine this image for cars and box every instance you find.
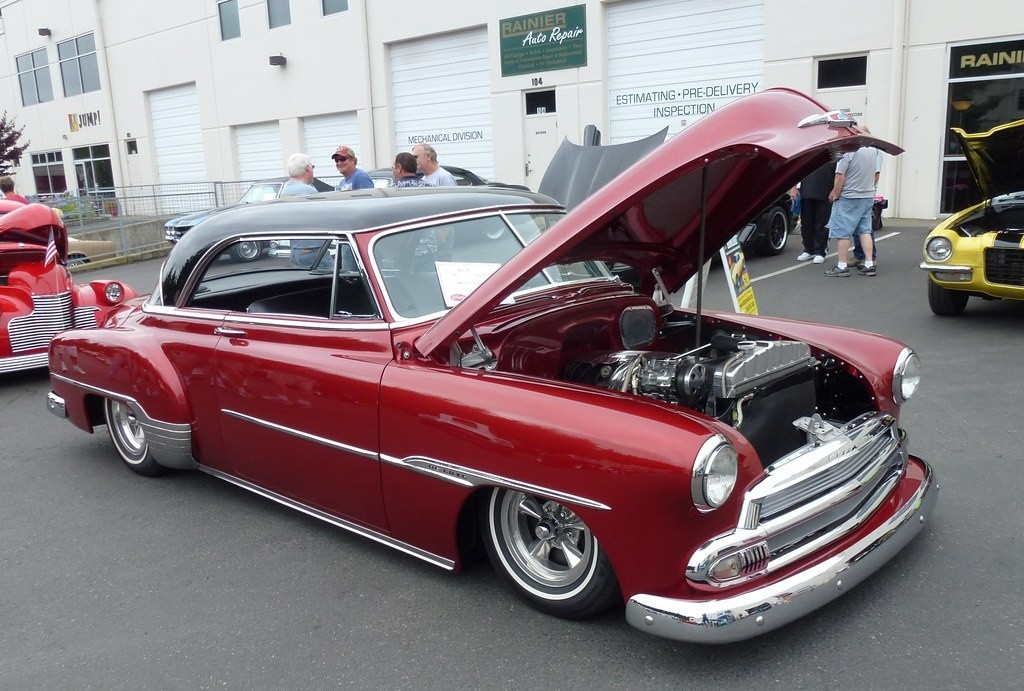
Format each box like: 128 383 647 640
0 200 138 377
163 164 534 277
918 119 1024 316
65 236 116 269
42 84 940 646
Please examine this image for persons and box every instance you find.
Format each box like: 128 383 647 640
0 176 29 206
331 145 375 272
279 155 335 271
824 123 883 277
789 155 837 264
384 153 447 271
411 143 459 266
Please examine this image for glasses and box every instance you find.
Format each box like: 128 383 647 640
335 157 349 162
309 165 314 169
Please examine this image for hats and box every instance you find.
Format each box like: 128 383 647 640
332 146 355 159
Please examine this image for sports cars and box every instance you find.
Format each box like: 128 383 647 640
532 124 796 265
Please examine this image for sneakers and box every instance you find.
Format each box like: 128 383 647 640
813 255 826 263
858 266 876 276
797 252 815 261
847 257 864 267
824 266 849 277
857 260 877 268
